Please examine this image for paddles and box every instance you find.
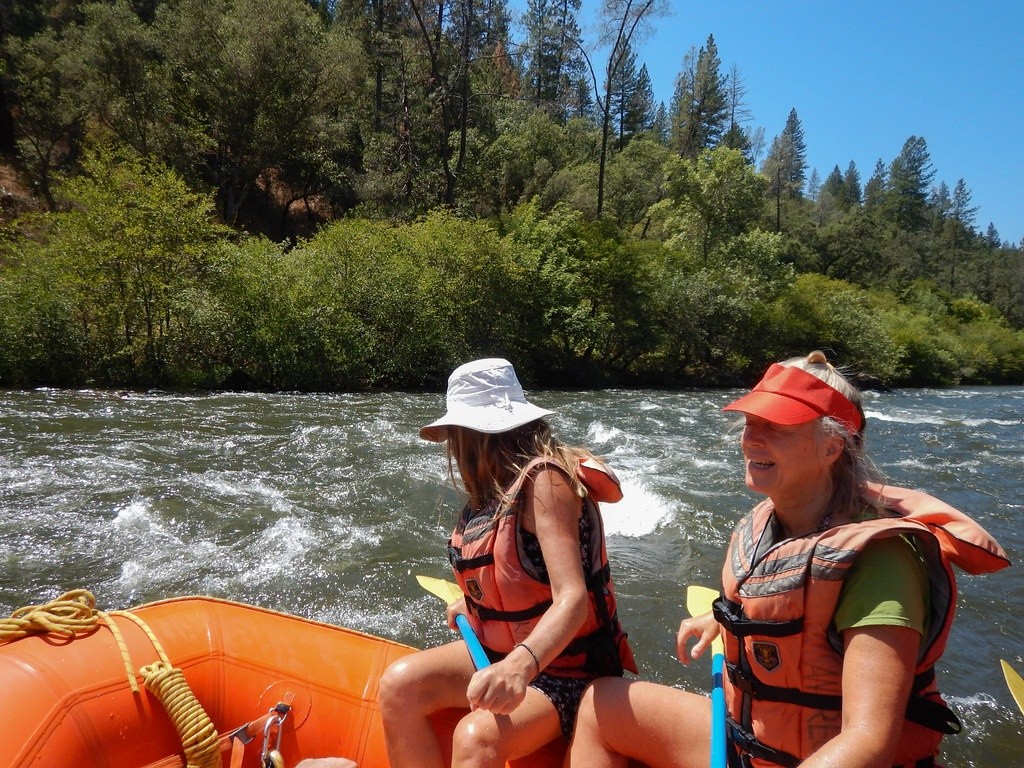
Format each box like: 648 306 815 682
999 657 1024 716
685 584 744 768
415 574 493 674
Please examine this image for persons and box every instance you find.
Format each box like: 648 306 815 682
378 358 625 768
563 351 957 768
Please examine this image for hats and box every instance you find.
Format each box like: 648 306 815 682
419 358 556 443
723 363 862 438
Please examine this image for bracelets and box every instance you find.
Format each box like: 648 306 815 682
515 643 540 682
